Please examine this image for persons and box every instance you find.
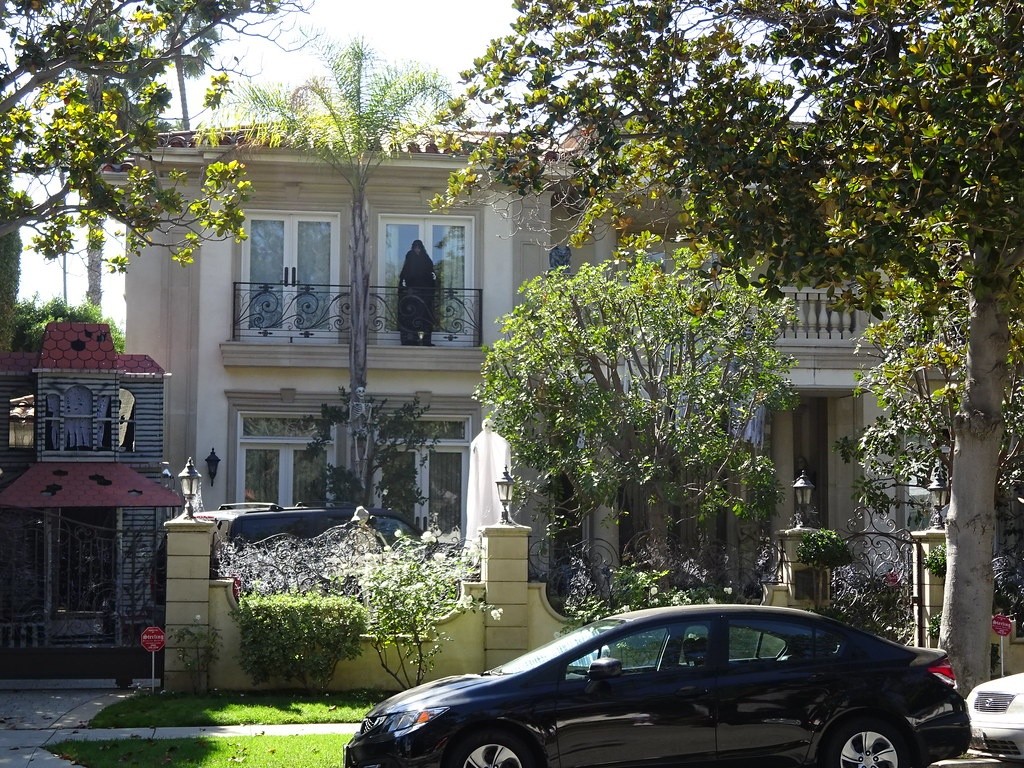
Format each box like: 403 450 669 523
794 455 817 497
398 239 436 347
345 506 378 553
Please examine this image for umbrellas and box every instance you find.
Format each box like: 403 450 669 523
460 418 511 566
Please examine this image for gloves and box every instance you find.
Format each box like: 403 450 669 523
432 272 437 280
402 279 407 288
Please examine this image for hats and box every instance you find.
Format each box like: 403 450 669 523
412 240 422 245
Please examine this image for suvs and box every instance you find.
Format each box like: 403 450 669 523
149 502 429 606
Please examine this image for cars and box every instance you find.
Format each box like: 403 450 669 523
344 603 973 768
965 673 1024 763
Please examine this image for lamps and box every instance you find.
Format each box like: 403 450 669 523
204 447 221 487
495 464 515 524
177 456 203 520
792 470 815 528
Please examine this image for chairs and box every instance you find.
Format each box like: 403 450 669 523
682 636 708 667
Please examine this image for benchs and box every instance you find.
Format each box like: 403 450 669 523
776 653 792 661
